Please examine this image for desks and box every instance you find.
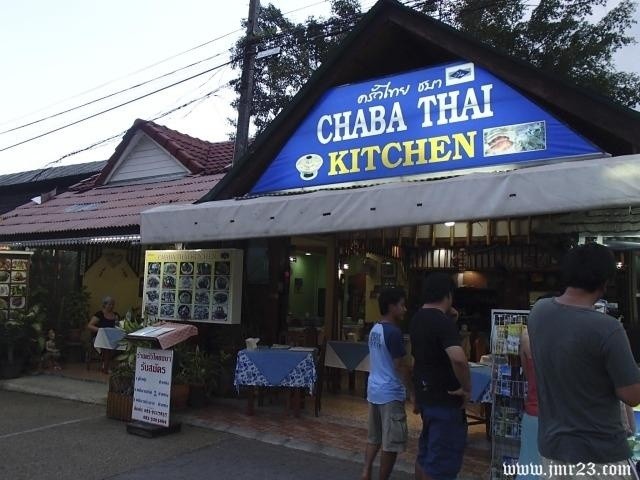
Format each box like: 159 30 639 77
234 324 369 419
465 362 492 439
93 328 125 374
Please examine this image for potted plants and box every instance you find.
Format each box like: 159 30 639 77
63 285 92 342
0 303 50 378
106 311 232 422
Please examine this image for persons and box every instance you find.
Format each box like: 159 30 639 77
516 263 569 479
525 240 639 479
87 293 121 376
411 272 476 479
359 284 421 480
36 328 62 373
